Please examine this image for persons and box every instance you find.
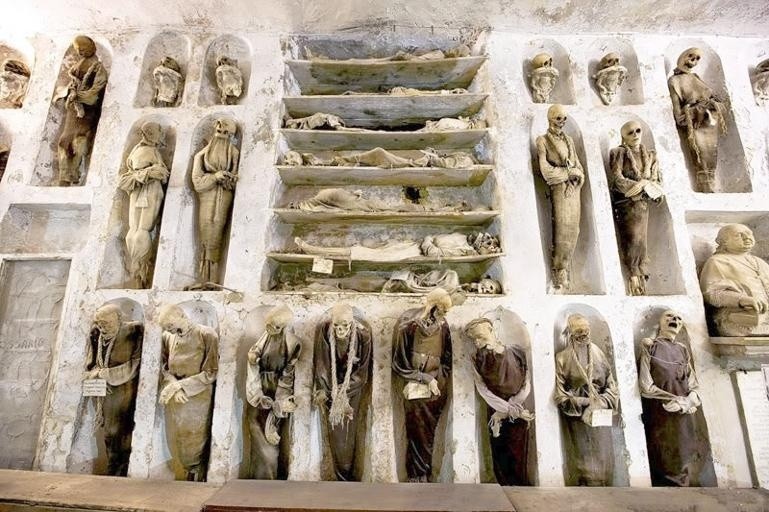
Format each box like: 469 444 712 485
699 222 769 338
